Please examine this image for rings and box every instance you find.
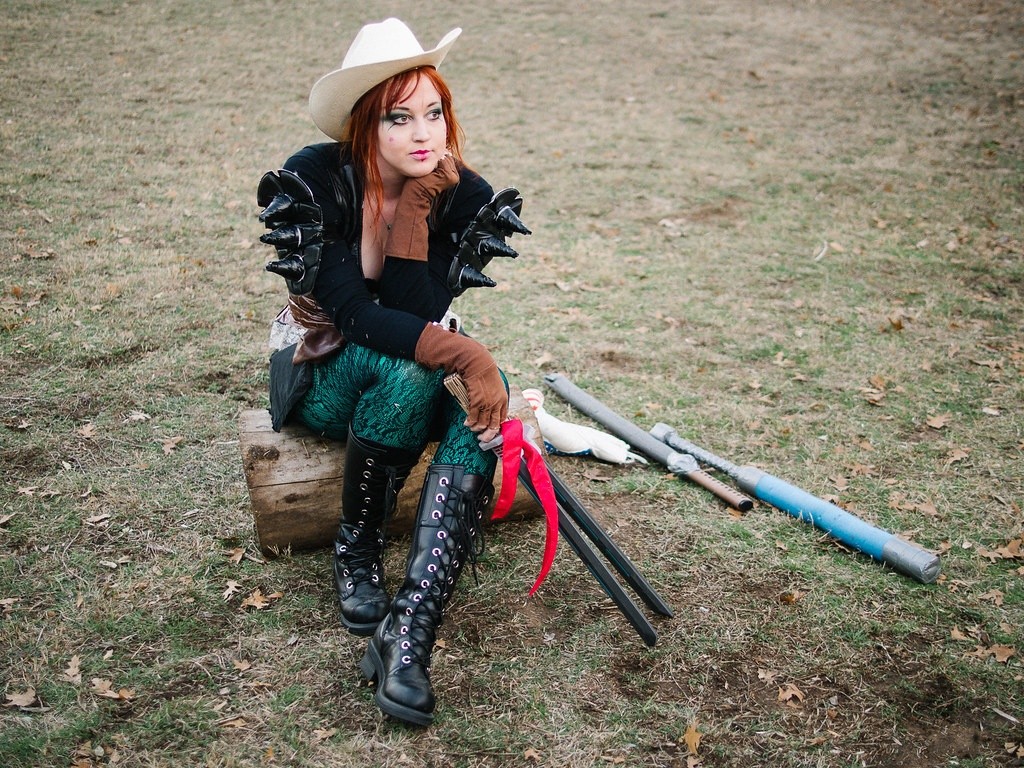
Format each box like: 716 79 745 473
488 426 500 430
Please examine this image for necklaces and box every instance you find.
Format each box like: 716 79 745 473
366 178 393 230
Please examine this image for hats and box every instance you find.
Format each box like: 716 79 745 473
309 18 464 142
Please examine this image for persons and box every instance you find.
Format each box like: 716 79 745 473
256 16 533 728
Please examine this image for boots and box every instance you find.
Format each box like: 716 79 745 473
357 460 498 728
329 422 426 639
